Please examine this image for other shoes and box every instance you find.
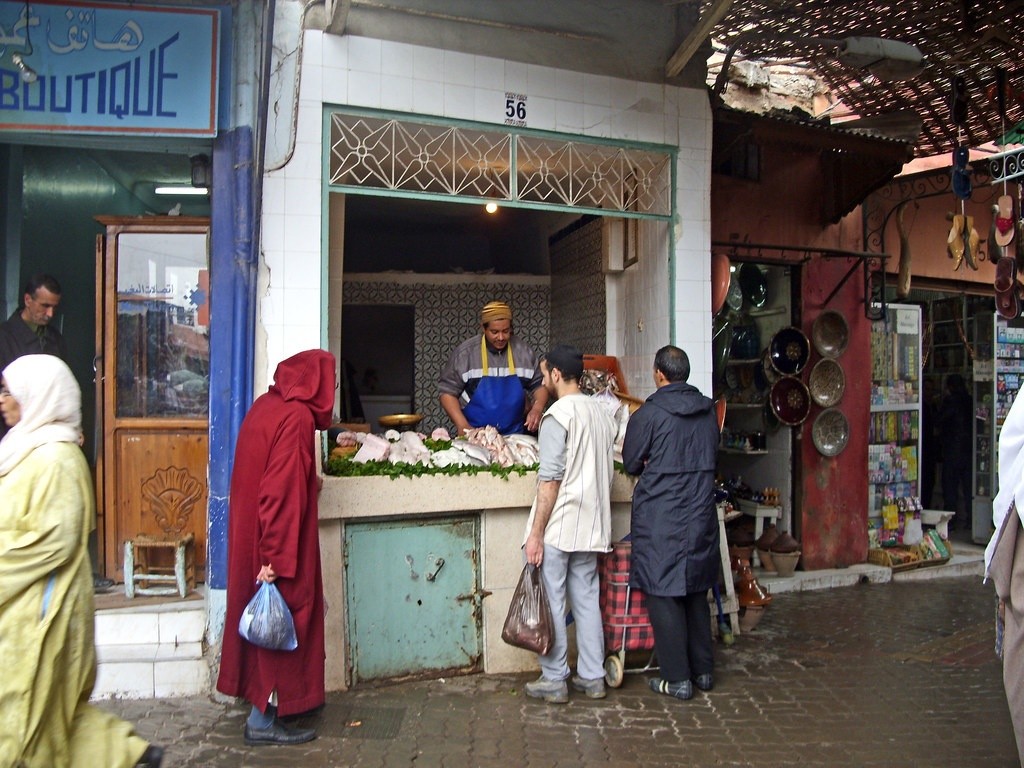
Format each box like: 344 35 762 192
136 743 163 768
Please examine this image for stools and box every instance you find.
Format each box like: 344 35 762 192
125 532 196 599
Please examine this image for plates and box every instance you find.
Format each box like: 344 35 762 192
812 409 850 457
812 310 850 358
760 348 782 383
770 378 812 425
809 359 845 408
713 364 767 404
761 388 780 432
767 327 810 378
711 254 768 317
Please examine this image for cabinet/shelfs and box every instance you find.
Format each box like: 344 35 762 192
925 295 995 517
870 300 922 529
720 306 787 457
972 308 1024 546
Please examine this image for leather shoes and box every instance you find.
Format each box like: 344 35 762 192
91 574 114 593
244 716 315 745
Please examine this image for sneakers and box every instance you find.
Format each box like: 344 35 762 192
649 677 692 700
693 672 714 690
572 674 605 698
523 674 569 702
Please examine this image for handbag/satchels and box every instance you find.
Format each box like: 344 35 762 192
238 578 297 651
500 562 555 656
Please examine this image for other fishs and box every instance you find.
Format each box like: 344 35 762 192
614 404 630 444
328 424 538 471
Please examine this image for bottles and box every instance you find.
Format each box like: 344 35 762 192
732 326 759 358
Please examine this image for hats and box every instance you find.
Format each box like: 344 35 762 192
482 301 512 325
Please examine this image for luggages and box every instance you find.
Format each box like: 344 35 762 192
599 539 665 688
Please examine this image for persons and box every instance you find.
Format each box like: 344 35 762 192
521 345 619 704
218 349 337 747
0 354 163 768
621 345 721 700
438 301 550 437
0 274 114 592
